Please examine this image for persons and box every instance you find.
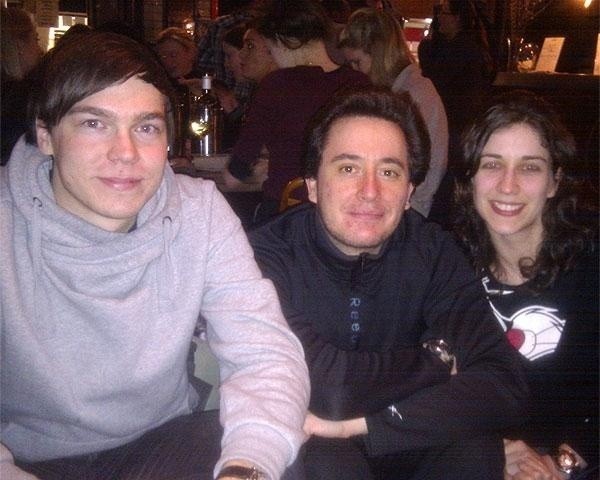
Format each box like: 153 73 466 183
1 0 600 480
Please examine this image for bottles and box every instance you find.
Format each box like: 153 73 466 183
191 73 221 155
